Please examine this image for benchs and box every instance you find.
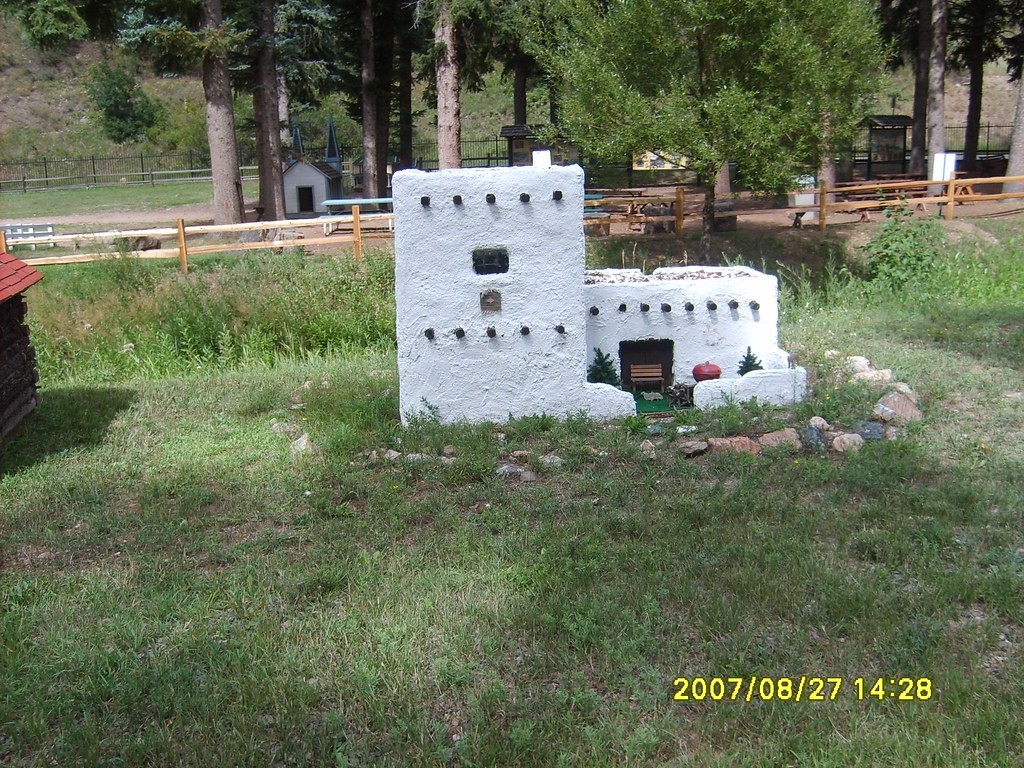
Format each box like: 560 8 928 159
584 194 662 211
253 206 264 222
835 184 982 215
319 208 395 234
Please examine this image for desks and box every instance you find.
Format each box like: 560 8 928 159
835 178 912 213
585 187 648 197
320 197 393 233
872 170 968 196
